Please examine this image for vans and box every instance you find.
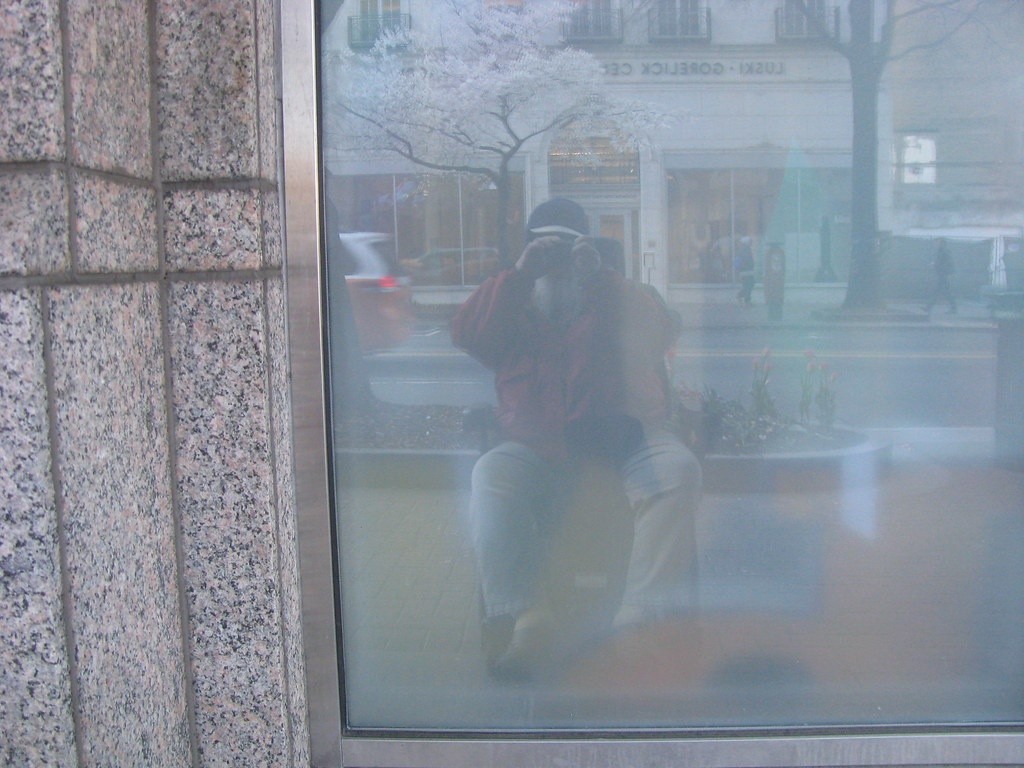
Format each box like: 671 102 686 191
405 246 502 277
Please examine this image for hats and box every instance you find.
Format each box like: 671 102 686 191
525 196 589 238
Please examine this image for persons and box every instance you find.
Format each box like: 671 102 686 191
445 199 699 680
920 236 957 315
734 235 785 322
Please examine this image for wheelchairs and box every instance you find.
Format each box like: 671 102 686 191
454 379 707 682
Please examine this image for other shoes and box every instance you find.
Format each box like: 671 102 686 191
491 615 545 670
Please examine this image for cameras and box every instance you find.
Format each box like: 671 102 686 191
543 236 581 257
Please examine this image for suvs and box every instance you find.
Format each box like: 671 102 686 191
337 231 412 302
